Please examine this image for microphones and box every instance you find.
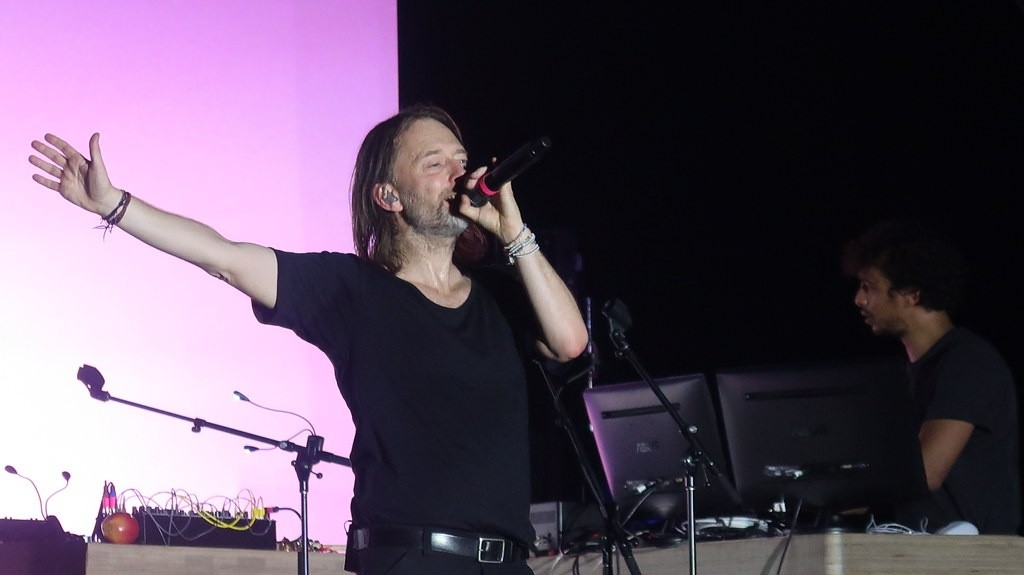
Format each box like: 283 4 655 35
465 136 552 207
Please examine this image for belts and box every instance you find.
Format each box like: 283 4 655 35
350 527 529 564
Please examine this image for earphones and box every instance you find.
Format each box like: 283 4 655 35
382 191 397 205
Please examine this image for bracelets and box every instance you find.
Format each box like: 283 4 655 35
504 224 541 266
95 189 131 241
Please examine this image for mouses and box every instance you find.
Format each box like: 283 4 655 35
938 522 979 537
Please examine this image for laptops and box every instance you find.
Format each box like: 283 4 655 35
583 373 746 528
717 361 932 534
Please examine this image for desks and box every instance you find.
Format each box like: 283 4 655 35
83 541 358 575
526 531 1024 574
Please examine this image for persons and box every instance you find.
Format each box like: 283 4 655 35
841 219 1021 534
26 105 593 575
